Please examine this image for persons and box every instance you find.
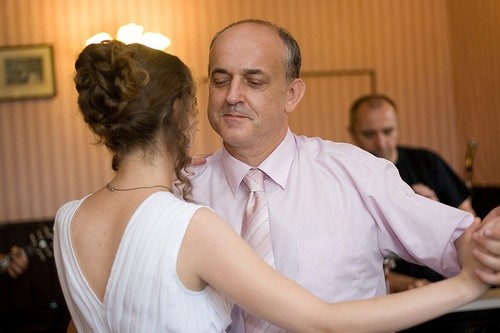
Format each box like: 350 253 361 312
172 19 500 333
54 40 500 333
345 95 476 292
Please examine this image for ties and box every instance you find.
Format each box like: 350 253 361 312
241 170 283 333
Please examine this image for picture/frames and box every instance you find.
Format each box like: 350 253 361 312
0 43 57 100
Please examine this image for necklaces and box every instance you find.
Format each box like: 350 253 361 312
106 185 173 193
